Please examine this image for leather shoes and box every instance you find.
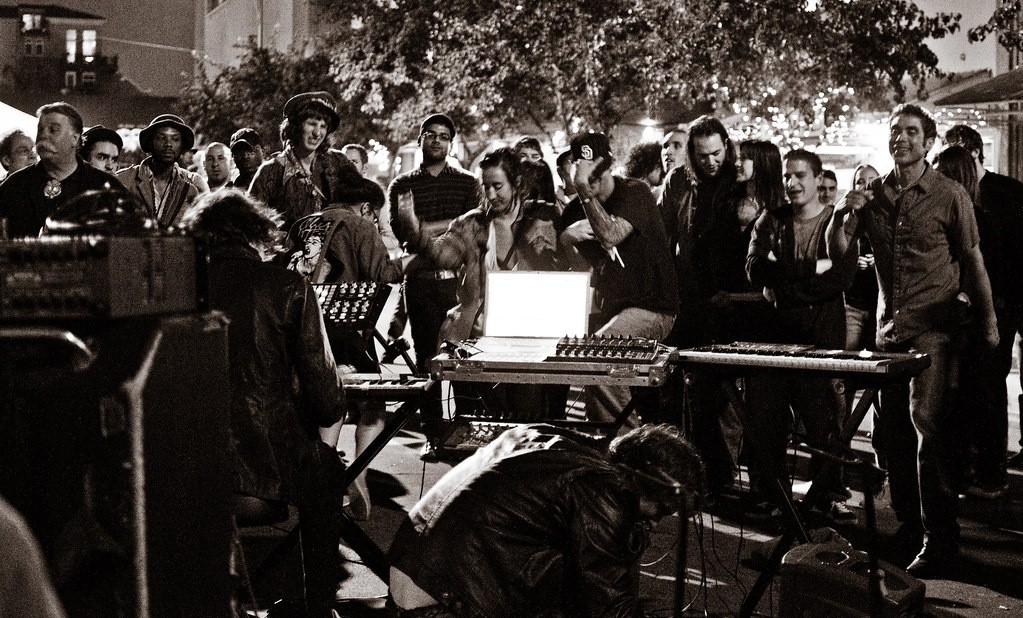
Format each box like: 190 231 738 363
904 533 963 576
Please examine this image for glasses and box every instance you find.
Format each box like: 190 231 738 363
422 132 452 143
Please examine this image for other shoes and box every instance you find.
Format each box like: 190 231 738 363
350 481 371 519
421 443 443 463
1006 449 1023 468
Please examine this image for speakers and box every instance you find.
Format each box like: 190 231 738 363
780 542 926 617
1 311 234 618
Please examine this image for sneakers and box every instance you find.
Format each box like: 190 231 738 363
818 501 858 525
969 475 1012 498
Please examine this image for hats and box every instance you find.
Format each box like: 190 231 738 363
571 131 614 164
283 89 340 133
230 127 263 150
140 114 195 156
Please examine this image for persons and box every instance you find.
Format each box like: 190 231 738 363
180 187 350 618
813 168 836 204
827 104 999 580
386 418 707 617
933 142 1015 499
0 83 782 515
747 152 860 528
835 160 897 471
936 124 1023 489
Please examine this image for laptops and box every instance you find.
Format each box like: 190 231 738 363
470 269 591 364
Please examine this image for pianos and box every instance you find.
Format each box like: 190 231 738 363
333 365 438 403
676 340 932 378
